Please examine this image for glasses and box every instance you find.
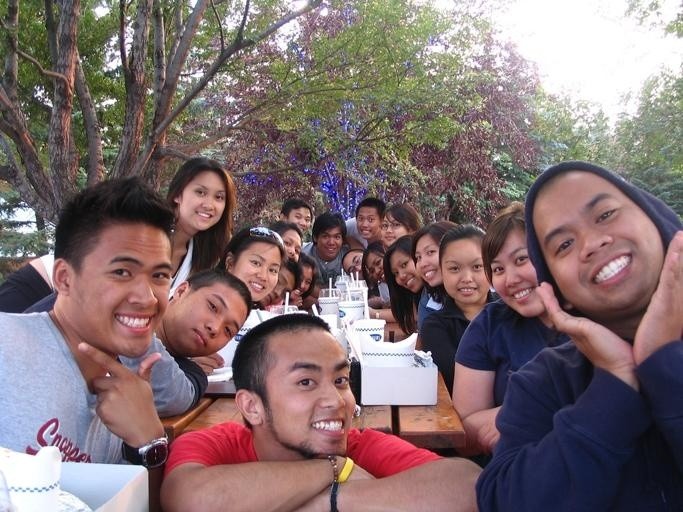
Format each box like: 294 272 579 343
379 223 403 229
249 226 284 249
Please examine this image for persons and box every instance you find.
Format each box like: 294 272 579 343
361 241 398 323
421 225 502 402
383 234 428 334
452 203 585 458
279 198 313 235
342 248 365 280
301 212 351 289
474 162 683 512
289 251 320 307
160 313 484 512
113 267 252 419
410 221 460 351
250 257 304 310
1 157 236 314
379 203 422 248
0 176 195 512
267 221 303 263
355 197 385 245
215 227 285 302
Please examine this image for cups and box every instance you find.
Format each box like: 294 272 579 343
318 296 338 313
351 290 362 300
336 282 346 294
338 300 365 325
355 319 385 343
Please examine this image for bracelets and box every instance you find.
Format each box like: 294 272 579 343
337 455 355 485
327 454 339 484
328 482 341 512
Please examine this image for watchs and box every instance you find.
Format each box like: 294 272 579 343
121 432 173 470
